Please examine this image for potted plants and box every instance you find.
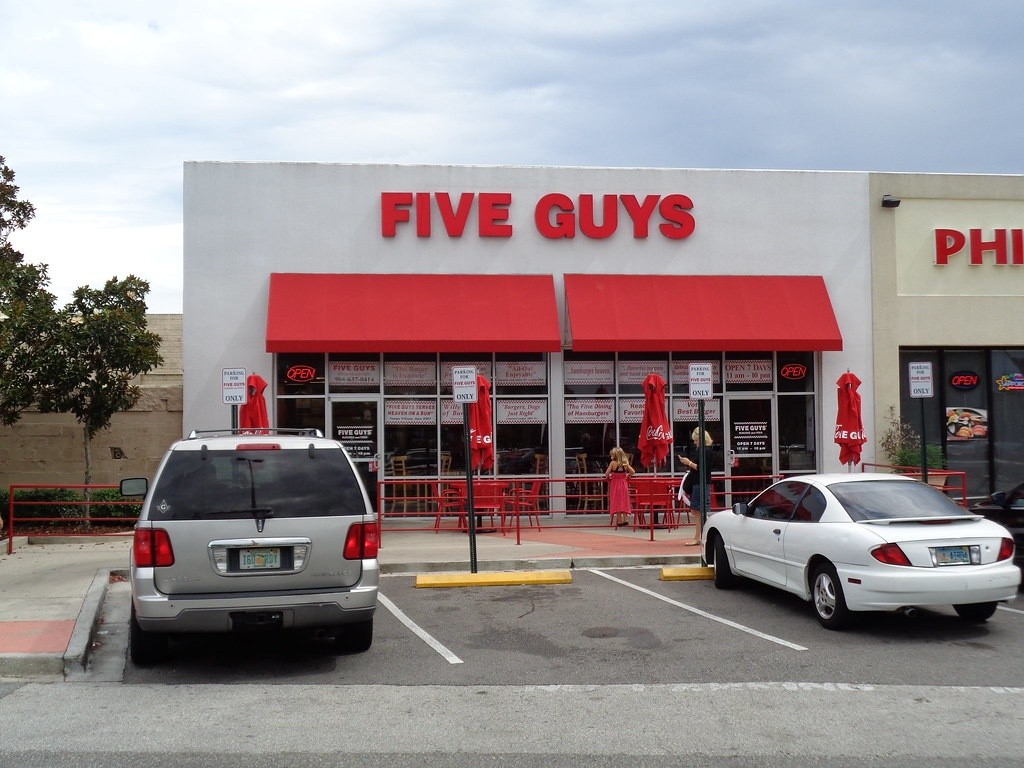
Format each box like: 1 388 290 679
890 445 952 492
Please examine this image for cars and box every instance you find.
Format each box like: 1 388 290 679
699 472 1024 631
969 480 1024 568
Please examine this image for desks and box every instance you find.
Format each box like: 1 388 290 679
629 480 681 529
450 482 509 532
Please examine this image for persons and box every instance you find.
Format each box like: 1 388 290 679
581 433 593 504
681 428 713 546
606 447 635 527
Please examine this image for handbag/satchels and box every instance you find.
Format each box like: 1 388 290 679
683 472 694 496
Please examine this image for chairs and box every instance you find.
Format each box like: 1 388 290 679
504 482 541 532
431 483 468 534
662 497 691 524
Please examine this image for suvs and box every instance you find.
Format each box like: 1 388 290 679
119 422 379 665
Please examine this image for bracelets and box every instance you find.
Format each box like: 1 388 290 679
689 462 692 466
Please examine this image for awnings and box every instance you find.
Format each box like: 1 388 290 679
266 272 562 352
564 273 843 351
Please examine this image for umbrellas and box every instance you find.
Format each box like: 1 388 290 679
239 372 269 434
638 371 673 523
834 369 867 473
470 373 494 527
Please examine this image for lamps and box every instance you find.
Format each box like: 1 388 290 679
880 194 901 208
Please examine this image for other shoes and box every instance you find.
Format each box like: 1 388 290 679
685 538 701 545
617 522 628 527
